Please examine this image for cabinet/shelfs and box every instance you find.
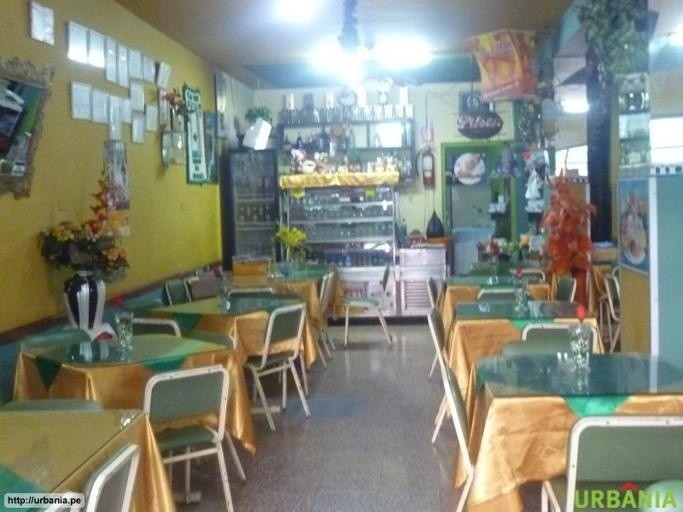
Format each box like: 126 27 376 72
277 118 416 186
287 185 395 320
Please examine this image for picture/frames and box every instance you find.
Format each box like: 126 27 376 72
0 56 56 200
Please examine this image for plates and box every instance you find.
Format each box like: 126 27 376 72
454 152 486 185
622 213 646 265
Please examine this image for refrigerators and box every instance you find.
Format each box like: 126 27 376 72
228 148 278 266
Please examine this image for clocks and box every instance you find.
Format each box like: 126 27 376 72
337 89 357 107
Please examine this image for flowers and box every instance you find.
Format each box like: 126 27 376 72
37 181 128 282
275 225 308 258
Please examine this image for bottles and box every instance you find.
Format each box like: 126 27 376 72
232 102 412 266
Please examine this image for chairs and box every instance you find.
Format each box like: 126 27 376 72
341 263 393 348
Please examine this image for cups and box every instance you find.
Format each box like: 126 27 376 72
568 322 592 373
512 274 530 312
114 311 134 352
216 279 231 311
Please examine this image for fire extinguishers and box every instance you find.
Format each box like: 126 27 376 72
415 146 435 186
